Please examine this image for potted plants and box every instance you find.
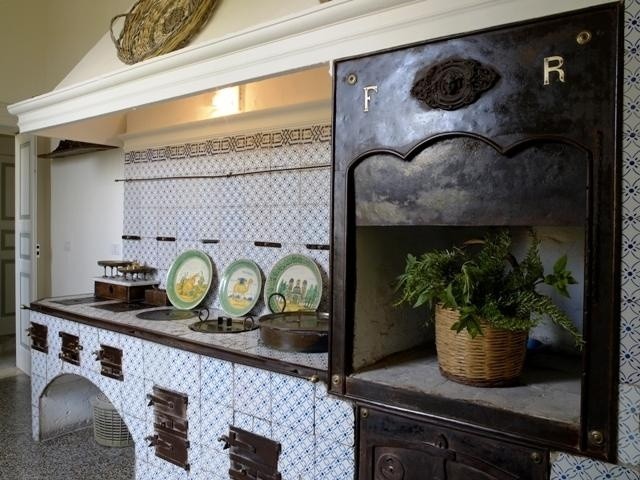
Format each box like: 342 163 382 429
383 229 586 389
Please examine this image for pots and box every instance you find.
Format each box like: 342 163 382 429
256 292 328 353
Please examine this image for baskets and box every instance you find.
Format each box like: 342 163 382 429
110 0 217 65
90 394 134 448
434 239 529 388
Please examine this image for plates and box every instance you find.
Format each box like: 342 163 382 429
219 259 262 316
264 253 323 316
166 249 213 311
